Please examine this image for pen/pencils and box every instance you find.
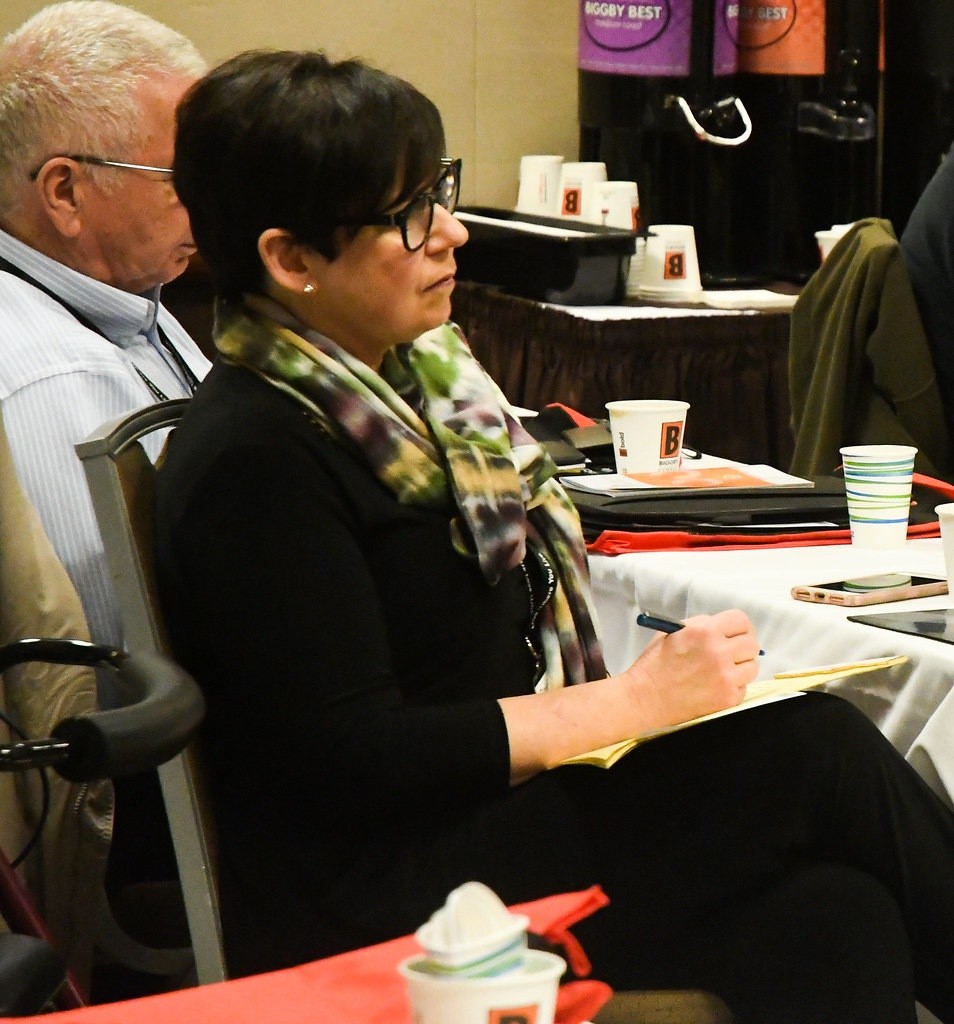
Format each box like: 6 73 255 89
636 610 765 656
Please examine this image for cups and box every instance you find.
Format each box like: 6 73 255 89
556 162 608 221
637 225 703 300
840 446 918 545
516 156 563 216
398 948 566 1024
816 230 847 263
589 183 645 295
935 503 954 602
606 399 690 474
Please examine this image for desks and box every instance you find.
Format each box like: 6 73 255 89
451 260 803 472
511 404 954 810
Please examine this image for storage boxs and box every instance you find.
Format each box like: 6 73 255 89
455 206 636 306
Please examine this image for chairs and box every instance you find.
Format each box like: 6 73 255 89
788 216 954 522
74 397 737 1024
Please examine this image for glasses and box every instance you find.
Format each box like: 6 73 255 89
316 157 464 251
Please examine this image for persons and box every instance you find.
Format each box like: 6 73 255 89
138 46 954 1024
2 1 210 738
900 140 954 415
